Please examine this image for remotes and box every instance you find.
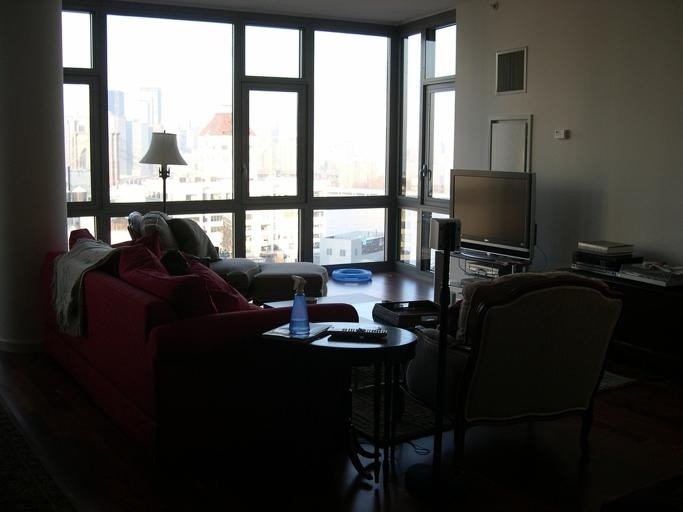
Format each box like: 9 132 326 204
326 327 388 337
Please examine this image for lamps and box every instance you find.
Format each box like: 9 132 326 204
138 130 186 212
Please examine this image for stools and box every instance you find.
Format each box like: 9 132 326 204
254 262 328 304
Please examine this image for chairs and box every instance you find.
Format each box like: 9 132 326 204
126 210 258 303
406 269 625 469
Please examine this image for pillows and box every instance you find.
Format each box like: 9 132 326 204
67 219 253 316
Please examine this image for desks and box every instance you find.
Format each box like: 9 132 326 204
260 318 418 483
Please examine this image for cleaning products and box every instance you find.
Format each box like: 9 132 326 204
289 273 310 338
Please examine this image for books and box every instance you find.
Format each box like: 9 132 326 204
575 239 634 256
261 320 332 343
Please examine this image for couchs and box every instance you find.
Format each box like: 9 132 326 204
43 253 360 467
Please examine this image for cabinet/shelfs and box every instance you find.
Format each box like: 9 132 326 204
433 253 524 303
564 267 683 376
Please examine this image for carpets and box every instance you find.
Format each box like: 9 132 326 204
1 398 79 510
343 368 636 449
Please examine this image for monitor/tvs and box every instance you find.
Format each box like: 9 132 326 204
450 168 535 262
